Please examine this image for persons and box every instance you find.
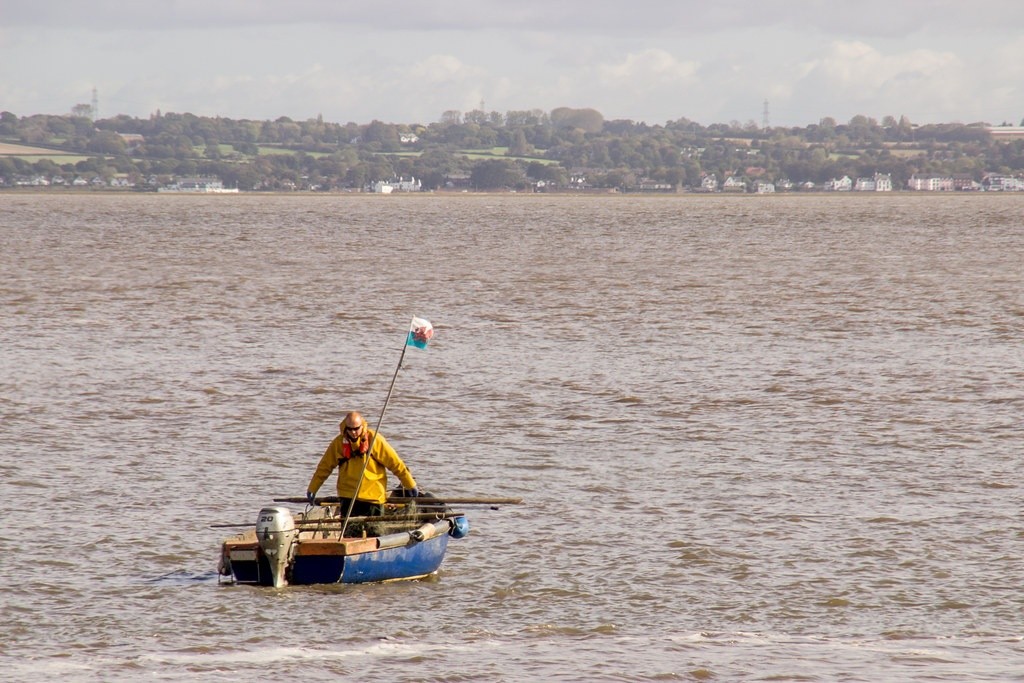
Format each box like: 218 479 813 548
307 412 418 537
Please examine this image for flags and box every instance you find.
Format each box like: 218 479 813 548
405 317 433 350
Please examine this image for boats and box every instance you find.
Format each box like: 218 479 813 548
217 476 467 588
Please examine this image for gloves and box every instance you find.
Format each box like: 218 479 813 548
307 491 315 506
410 486 418 497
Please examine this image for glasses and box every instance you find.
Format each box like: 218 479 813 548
346 425 361 431
